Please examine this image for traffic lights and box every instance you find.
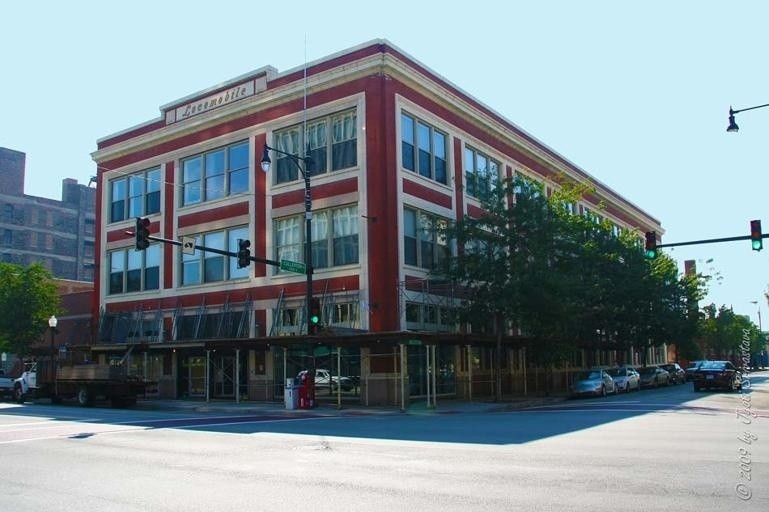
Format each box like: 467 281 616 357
308 297 320 336
236 238 251 270
133 216 151 251
750 219 763 251
645 231 657 259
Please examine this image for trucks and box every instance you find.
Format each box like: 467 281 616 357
20 343 157 409
0 369 17 401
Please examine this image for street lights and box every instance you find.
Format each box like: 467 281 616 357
260 141 316 409
48 314 58 406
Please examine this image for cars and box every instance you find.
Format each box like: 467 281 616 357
296 369 354 392
692 361 743 393
571 360 702 398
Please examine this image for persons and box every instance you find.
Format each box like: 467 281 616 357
24 357 37 372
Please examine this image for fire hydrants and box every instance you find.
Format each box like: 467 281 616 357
299 371 315 409
